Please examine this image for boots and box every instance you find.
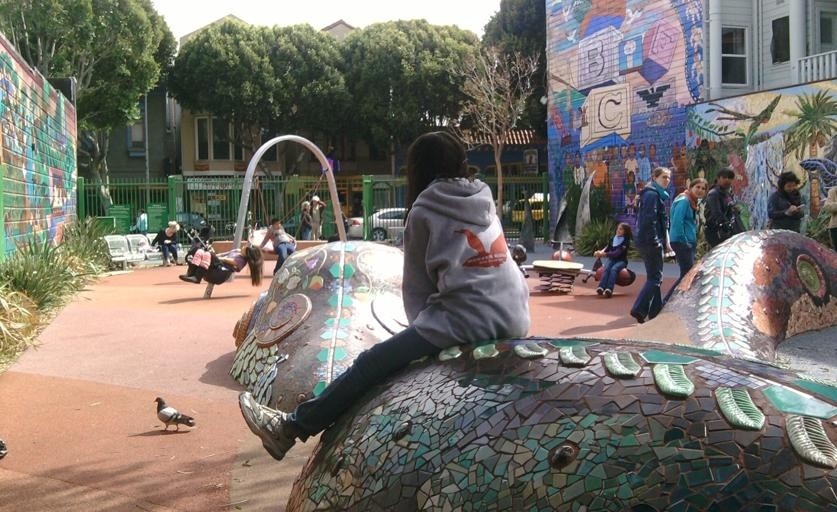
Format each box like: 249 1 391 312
179 264 207 283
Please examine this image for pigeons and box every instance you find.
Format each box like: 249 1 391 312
0 440 8 459
154 397 196 432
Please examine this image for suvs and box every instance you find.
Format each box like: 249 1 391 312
512 199 551 234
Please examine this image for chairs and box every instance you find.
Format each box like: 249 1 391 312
103 232 181 270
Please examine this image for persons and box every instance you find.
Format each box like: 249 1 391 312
258 217 295 278
310 195 326 242
128 209 148 235
178 242 263 287
662 178 709 307
562 142 688 217
626 167 669 329
765 171 804 234
703 167 741 248
300 201 311 239
150 219 183 268
592 221 633 297
821 184 836 254
235 130 532 465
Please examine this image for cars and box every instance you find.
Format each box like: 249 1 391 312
343 206 407 243
256 214 297 237
176 212 206 242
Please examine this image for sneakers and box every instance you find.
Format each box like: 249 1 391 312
239 391 296 460
597 287 612 296
